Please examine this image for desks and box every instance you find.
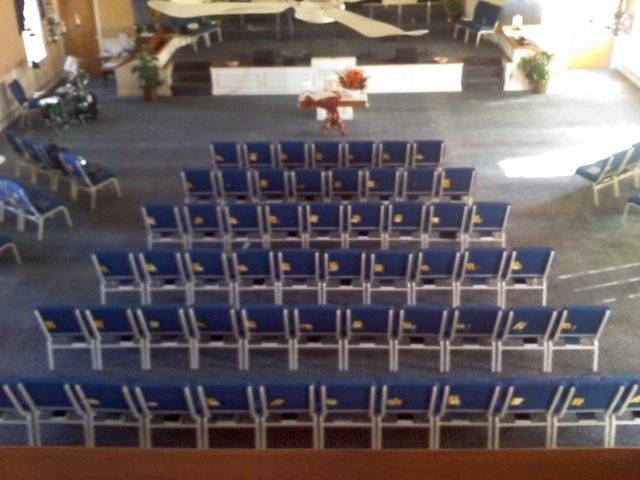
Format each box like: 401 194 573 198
496 25 545 94
298 100 370 140
209 53 463 97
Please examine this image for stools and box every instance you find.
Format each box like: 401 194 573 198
102 59 130 90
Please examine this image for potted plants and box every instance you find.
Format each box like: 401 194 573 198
521 51 553 94
131 45 165 99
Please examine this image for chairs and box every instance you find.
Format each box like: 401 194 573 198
255 48 279 57
453 1 503 48
141 201 515 246
175 15 222 49
209 137 447 167
89 247 557 305
396 48 418 57
32 302 612 373
2 130 125 271
1 372 640 451
6 80 48 131
572 130 638 227
178 162 479 204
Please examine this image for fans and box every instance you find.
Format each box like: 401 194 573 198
144 0 430 38
